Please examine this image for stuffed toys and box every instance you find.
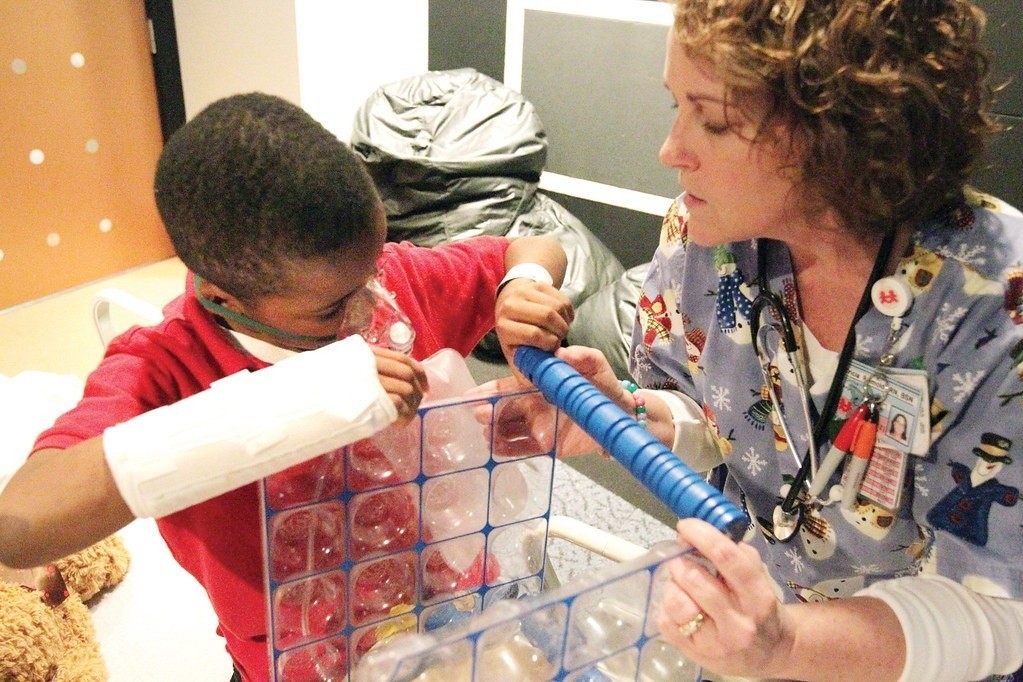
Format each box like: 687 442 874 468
1 531 131 682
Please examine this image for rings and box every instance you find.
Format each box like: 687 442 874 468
680 611 709 638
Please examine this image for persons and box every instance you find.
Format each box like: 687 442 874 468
462 0 1023 682
886 414 909 446
1 92 576 682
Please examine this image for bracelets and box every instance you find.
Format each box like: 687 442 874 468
493 261 554 300
596 380 648 463
